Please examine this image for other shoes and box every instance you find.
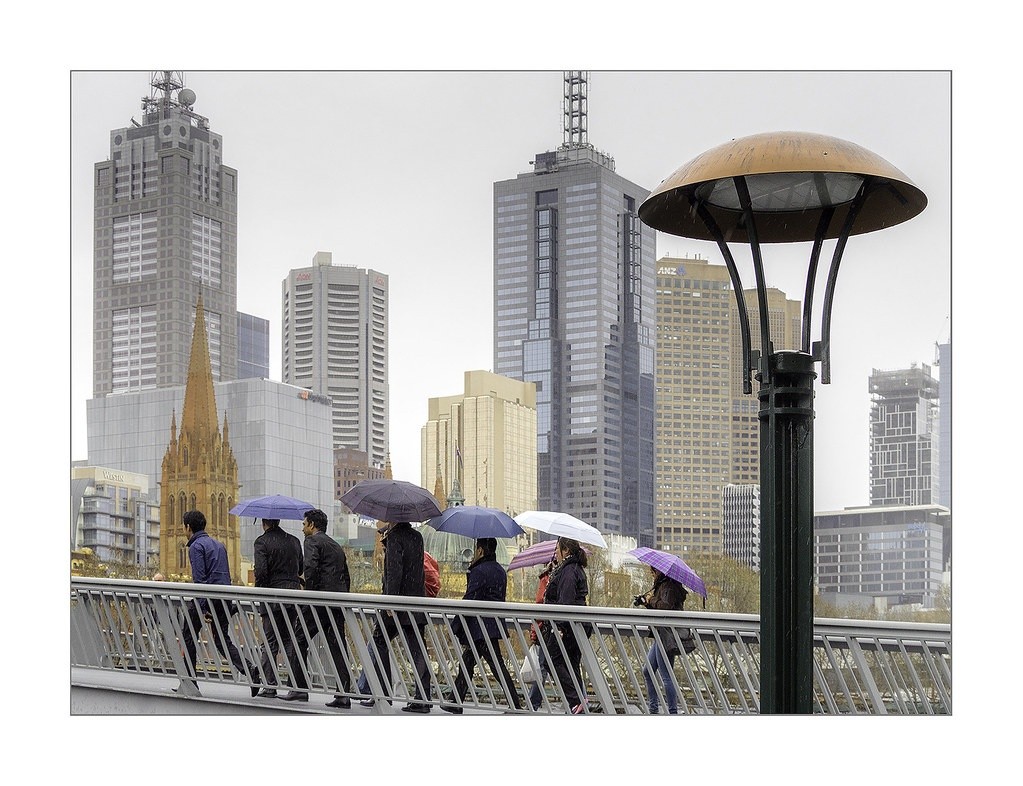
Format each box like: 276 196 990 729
439 704 464 714
360 698 393 707
401 702 431 713
256 681 277 698
171 685 200 693
407 702 434 708
574 698 589 714
249 664 260 698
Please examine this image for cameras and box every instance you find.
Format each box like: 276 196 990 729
633 595 646 607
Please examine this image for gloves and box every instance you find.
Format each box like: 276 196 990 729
634 594 647 605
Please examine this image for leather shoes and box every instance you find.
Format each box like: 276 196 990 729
325 697 351 709
275 690 309 702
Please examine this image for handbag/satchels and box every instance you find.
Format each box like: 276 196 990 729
519 643 544 683
535 560 558 603
659 627 696 655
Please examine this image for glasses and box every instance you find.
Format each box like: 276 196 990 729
652 568 657 574
557 536 567 549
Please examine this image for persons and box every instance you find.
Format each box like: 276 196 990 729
171 510 260 697
634 565 686 714
352 521 434 714
440 538 524 714
277 509 351 709
522 537 589 714
254 518 303 697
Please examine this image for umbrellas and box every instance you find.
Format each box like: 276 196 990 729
339 479 443 522
513 510 608 573
627 547 708 606
507 539 593 573
424 505 528 561
229 493 316 520
